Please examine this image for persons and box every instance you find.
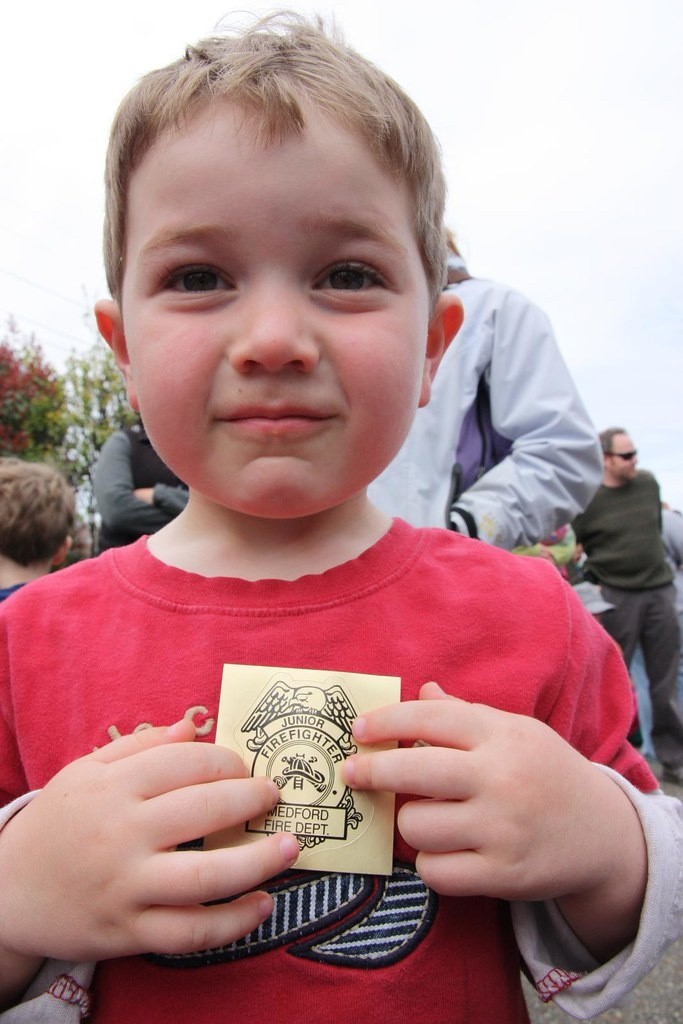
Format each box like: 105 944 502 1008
511 428 683 787
94 418 189 558
0 457 76 604
1 31 683 1023
366 232 606 552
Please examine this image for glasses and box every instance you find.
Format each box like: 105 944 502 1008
610 450 637 461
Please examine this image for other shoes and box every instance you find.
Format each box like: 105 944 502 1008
662 766 683 785
